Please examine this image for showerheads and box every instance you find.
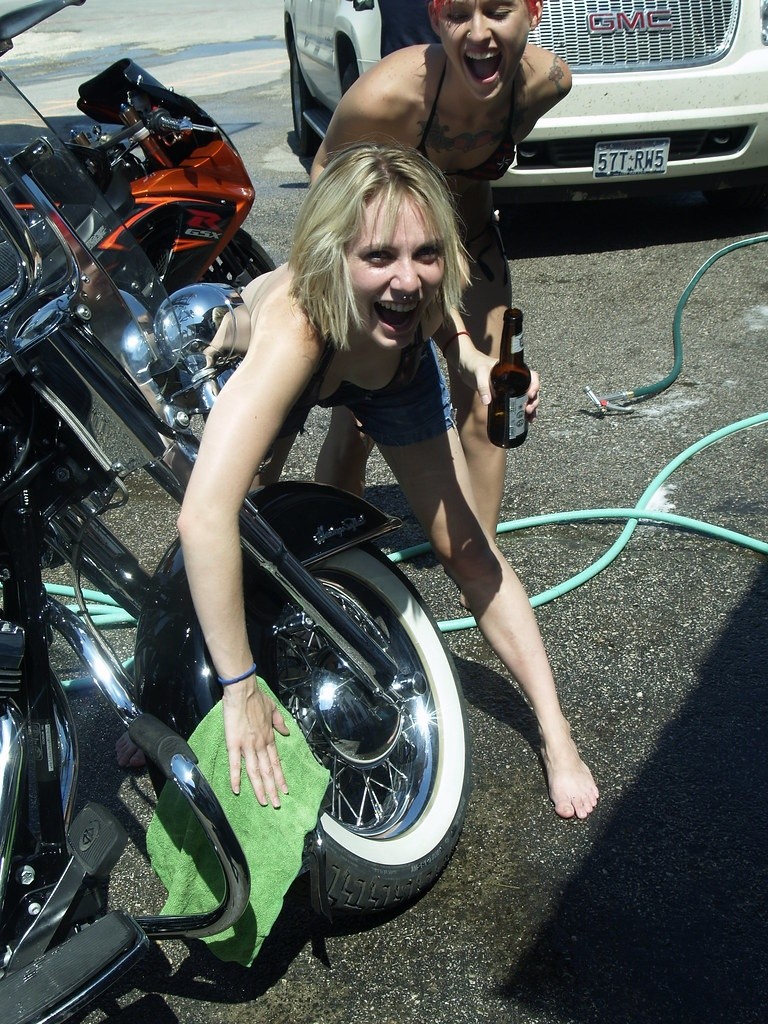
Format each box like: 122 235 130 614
582 385 635 416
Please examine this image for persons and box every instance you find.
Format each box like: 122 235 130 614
174 138 599 821
310 0 573 609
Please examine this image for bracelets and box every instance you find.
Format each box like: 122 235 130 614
218 664 258 685
442 331 470 358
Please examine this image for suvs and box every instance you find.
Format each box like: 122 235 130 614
281 0 768 205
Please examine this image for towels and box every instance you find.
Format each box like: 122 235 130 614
144 674 331 968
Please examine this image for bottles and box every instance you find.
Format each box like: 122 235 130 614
484 308 531 449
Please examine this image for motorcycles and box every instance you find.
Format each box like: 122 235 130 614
0 0 471 1023
